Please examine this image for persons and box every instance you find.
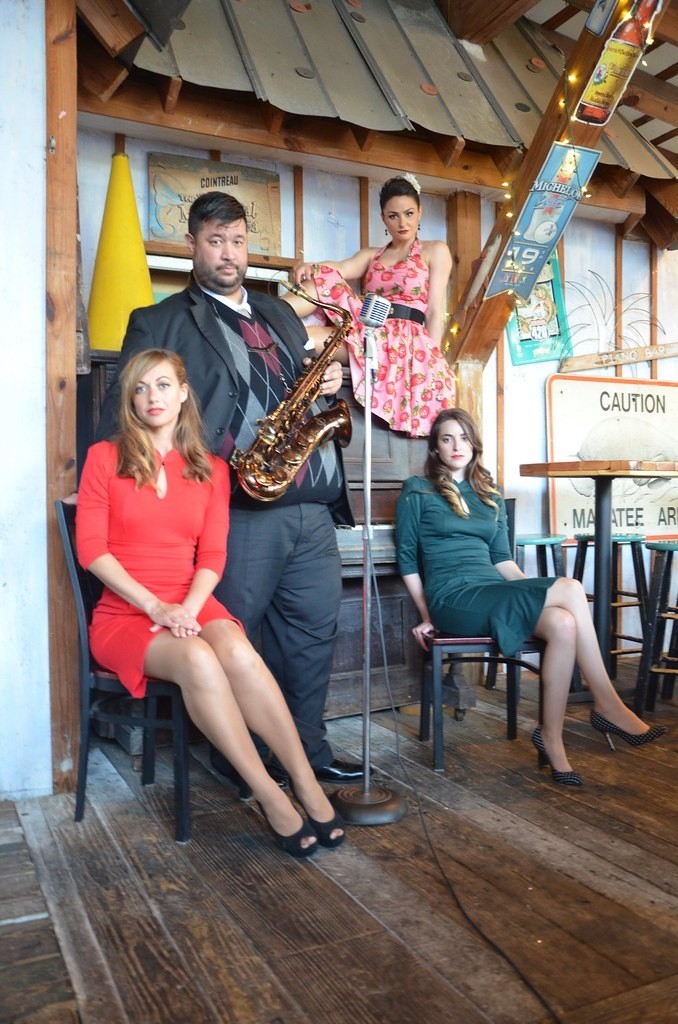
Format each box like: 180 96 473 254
74 350 346 856
394 408 667 784
64 193 374 797
281 175 456 438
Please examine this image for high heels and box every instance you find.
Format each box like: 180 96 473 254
533 725 584 787
590 706 668 752
287 773 346 849
255 796 318 861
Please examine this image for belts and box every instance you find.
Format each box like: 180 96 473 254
383 303 424 323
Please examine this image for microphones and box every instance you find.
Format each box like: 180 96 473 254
359 291 391 382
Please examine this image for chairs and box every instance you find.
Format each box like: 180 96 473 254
419 498 552 773
53 500 245 847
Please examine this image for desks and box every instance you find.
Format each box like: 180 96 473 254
520 459 678 704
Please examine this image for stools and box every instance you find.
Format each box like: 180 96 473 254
485 532 585 693
633 534 678 720
571 533 662 695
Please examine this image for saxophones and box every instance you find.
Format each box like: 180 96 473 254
228 279 354 502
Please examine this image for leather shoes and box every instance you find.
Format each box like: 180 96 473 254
209 754 290 793
299 760 377 783
